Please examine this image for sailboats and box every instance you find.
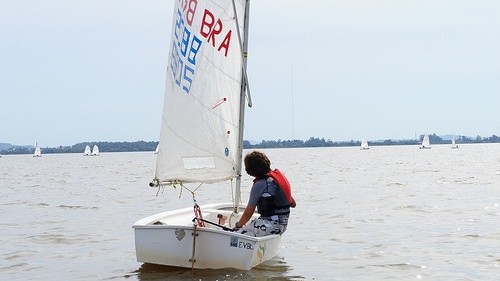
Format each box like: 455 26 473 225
152 141 159 154
132 0 287 271
450 135 459 149
32 139 43 157
419 133 431 150
92 145 100 157
82 144 91 156
359 136 370 151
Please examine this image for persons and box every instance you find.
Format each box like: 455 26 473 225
231 151 296 236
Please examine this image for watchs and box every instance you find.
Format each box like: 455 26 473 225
235 220 243 229
